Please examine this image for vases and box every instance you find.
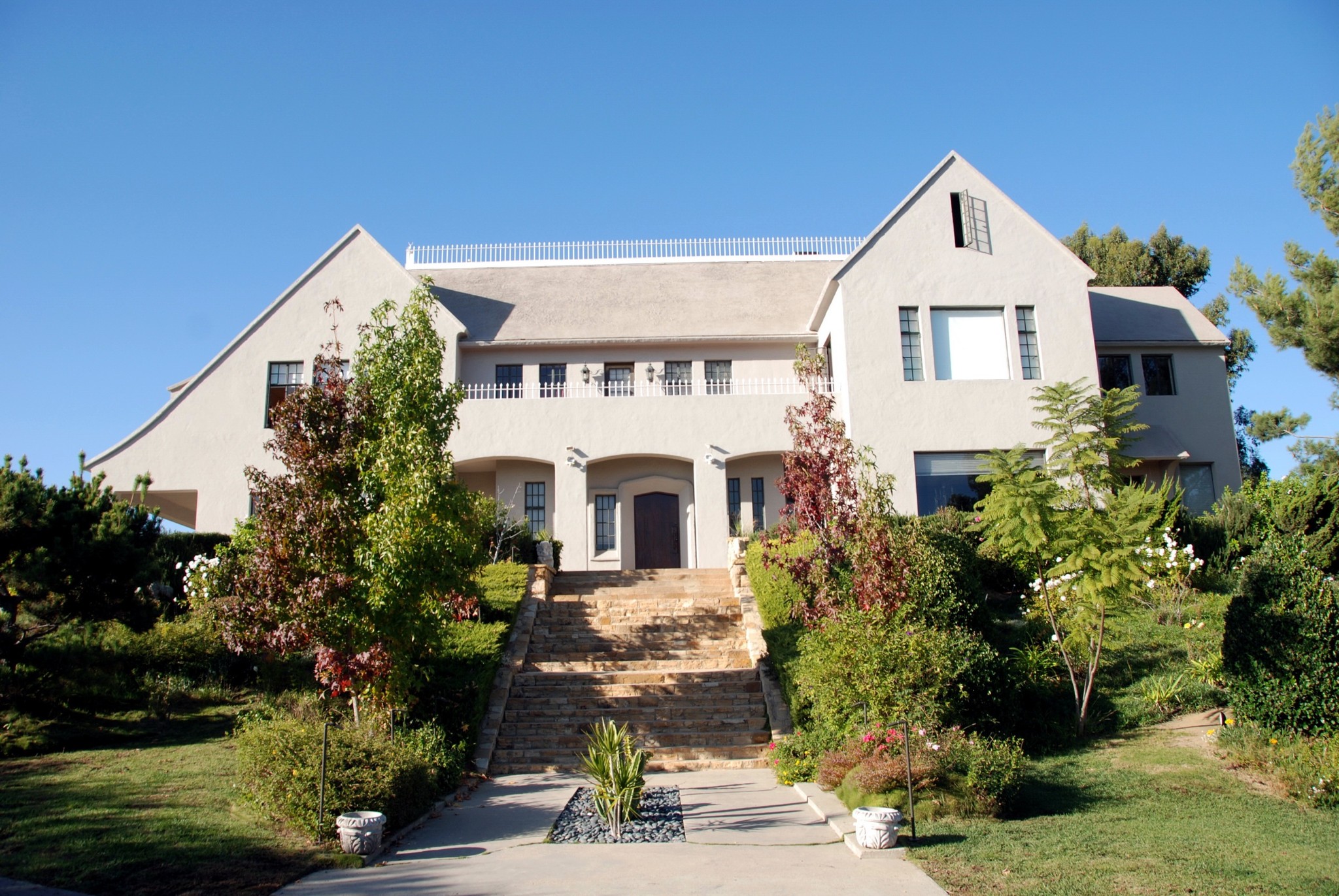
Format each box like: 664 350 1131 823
852 805 903 850
335 810 387 855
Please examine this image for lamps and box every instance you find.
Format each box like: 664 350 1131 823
643 358 657 383
579 360 591 386
